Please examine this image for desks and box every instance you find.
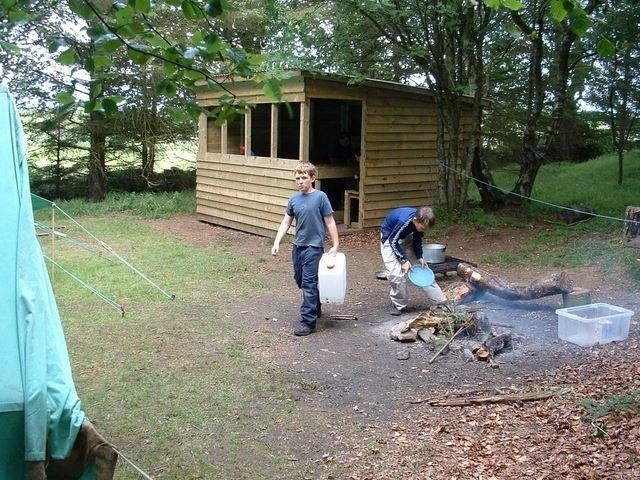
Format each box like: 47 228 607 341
314 163 352 189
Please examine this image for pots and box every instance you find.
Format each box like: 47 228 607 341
421 242 448 264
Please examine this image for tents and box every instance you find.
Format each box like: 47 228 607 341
0 80 120 480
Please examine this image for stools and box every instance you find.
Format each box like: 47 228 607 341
343 190 359 226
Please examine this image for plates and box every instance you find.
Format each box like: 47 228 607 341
408 264 436 287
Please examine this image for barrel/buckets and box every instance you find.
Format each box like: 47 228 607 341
317 252 347 306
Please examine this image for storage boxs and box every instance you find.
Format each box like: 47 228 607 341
555 302 635 347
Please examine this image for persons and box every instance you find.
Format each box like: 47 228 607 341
326 131 355 209
344 144 361 221
379 204 455 315
270 159 340 337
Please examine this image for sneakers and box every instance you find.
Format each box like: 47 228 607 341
389 301 400 316
295 320 317 336
